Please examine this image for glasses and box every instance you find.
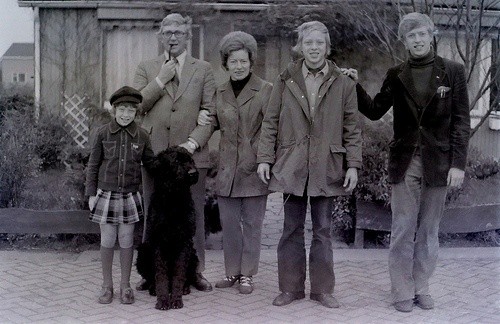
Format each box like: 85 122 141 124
162 30 187 38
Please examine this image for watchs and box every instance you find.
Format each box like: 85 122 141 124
187 141 197 150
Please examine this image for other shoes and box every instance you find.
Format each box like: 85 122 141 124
136 278 147 290
120 288 135 304
191 272 211 292
394 300 413 312
272 292 305 306
310 293 339 308
415 295 435 309
99 287 113 304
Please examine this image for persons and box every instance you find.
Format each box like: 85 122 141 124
340 11 471 313
197 30 274 294
131 12 217 294
255 21 363 309
84 85 154 305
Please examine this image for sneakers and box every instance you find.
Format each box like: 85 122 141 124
215 275 240 288
239 275 253 294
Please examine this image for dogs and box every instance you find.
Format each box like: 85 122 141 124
136 146 199 310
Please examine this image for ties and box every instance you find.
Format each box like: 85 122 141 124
172 59 180 98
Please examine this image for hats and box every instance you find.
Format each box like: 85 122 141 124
109 86 143 104
